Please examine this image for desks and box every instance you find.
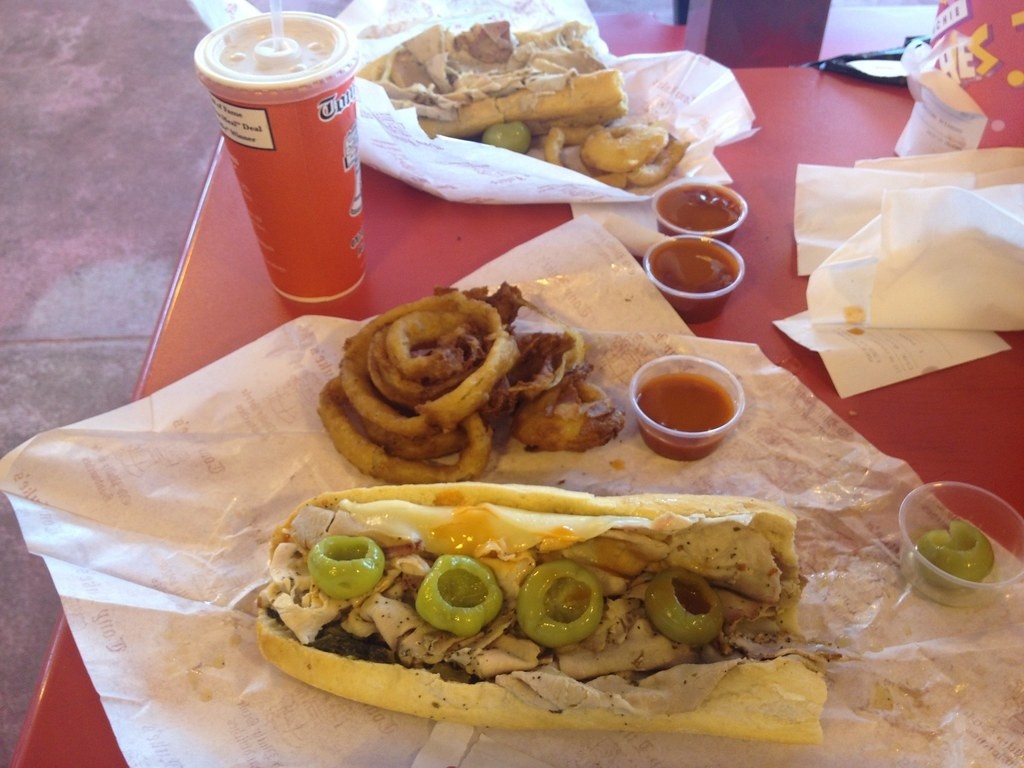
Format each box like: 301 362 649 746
28 0 1024 768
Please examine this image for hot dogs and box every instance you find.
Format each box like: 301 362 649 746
253 480 845 744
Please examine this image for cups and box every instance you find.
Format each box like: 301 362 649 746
193 11 368 304
629 355 746 462
896 481 1024 607
644 177 749 324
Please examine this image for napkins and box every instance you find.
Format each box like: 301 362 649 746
787 148 1024 332
